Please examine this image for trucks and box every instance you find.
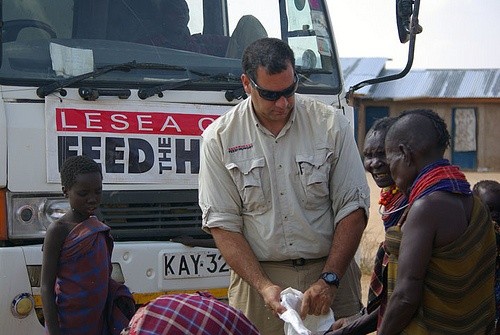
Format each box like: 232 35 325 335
0 0 423 335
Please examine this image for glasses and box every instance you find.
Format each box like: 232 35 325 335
245 70 298 101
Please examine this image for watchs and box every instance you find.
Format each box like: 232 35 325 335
318 271 340 289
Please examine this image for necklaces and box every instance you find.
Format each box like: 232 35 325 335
378 194 409 215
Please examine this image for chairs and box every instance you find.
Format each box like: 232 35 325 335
2 0 75 42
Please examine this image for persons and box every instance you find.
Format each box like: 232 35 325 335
321 109 500 335
198 37 371 335
40 156 136 335
